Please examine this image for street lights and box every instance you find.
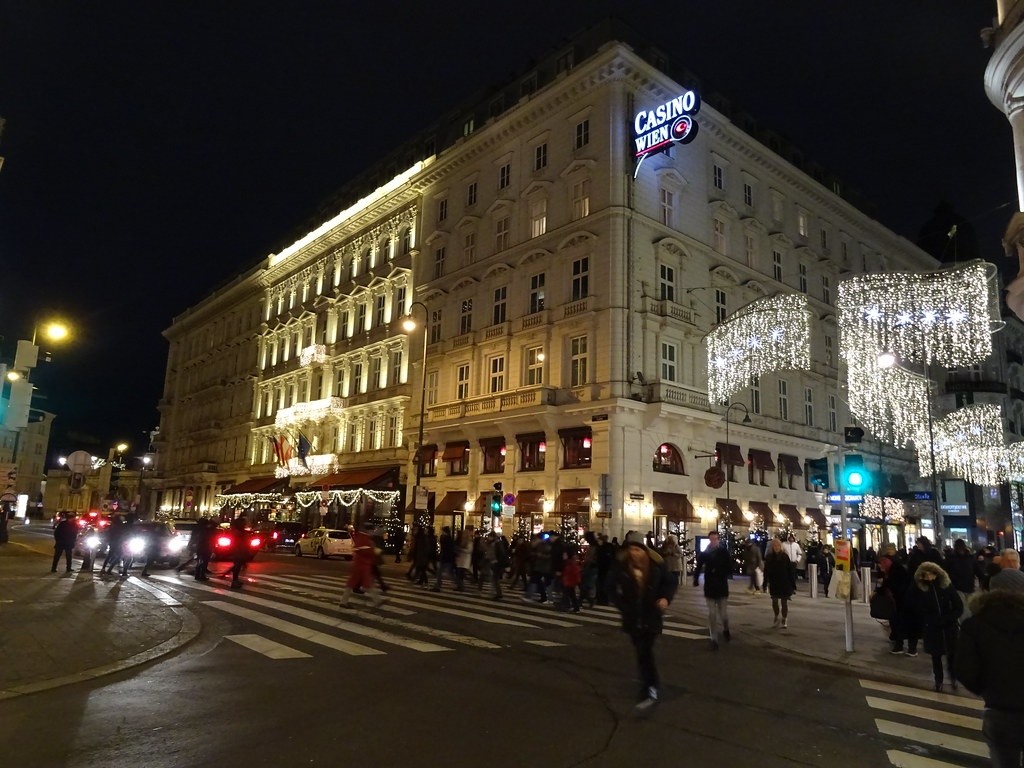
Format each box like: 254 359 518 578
2 321 65 521
404 302 429 536
726 402 752 526
118 444 127 495
877 323 940 544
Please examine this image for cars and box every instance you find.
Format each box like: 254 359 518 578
120 521 181 568
293 528 354 562
252 520 306 553
167 518 260 563
54 509 141 558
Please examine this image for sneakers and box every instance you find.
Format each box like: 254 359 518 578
906 648 918 657
889 645 904 654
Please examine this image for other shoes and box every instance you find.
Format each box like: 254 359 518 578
773 614 780 621
711 641 719 650
721 630 731 641
781 617 789 627
334 574 607 612
744 587 752 592
934 683 943 691
950 675 959 688
51 560 245 589
754 589 762 594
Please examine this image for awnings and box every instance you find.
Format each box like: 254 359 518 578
721 445 803 475
718 499 826 524
513 489 587 517
656 494 694 519
412 442 465 465
222 476 287 495
405 492 499 516
307 468 397 490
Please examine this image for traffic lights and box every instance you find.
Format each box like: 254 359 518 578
843 426 865 445
492 494 501 516
809 456 834 490
494 482 502 490
105 464 121 496
844 455 864 488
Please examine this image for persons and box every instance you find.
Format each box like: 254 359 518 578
175 514 247 588
394 527 404 561
339 523 390 608
694 532 734 650
101 515 165 577
852 536 1024 768
745 535 835 628
52 512 77 572
608 540 679 701
406 525 682 614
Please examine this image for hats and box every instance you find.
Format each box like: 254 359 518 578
989 568 1024 597
616 531 665 560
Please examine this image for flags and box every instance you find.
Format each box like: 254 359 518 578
269 432 311 469
